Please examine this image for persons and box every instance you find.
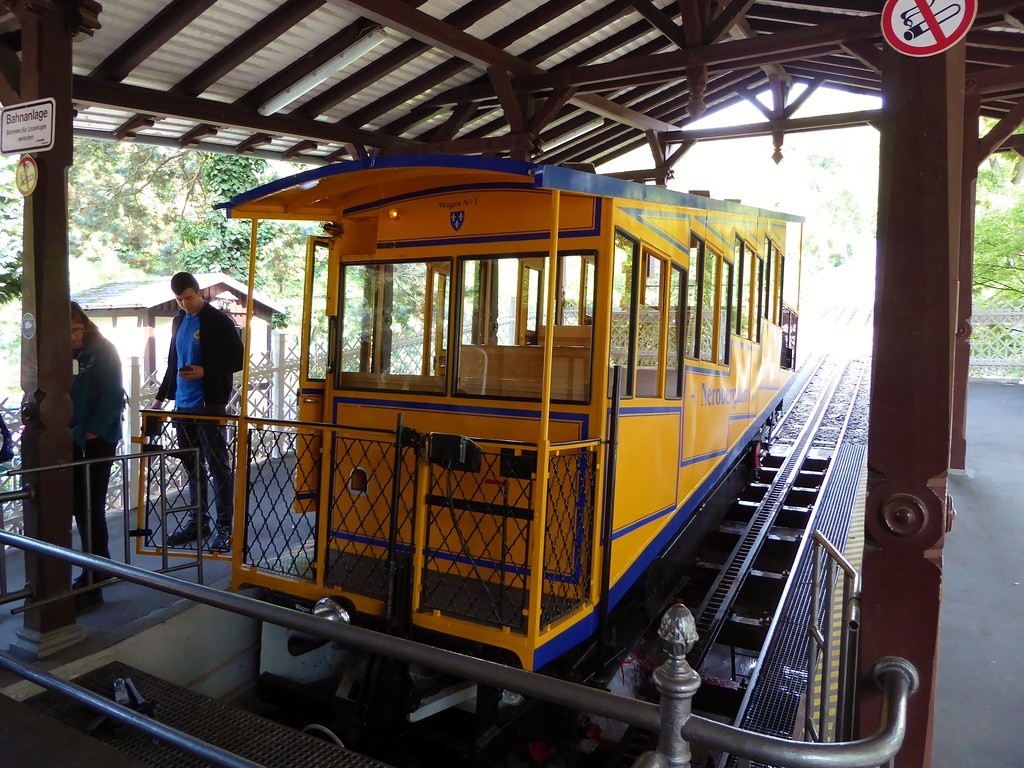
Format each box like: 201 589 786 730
69 301 123 591
150 272 244 551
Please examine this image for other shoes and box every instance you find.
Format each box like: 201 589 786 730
75 565 113 583
72 579 103 614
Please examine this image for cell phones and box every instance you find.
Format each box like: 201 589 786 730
178 366 193 372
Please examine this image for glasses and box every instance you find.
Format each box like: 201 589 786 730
72 326 85 336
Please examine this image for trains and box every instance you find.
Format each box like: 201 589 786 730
212 150 806 725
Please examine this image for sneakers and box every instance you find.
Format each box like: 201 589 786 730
165 520 210 546
209 524 231 551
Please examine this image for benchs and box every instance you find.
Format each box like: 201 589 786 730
341 305 727 404
